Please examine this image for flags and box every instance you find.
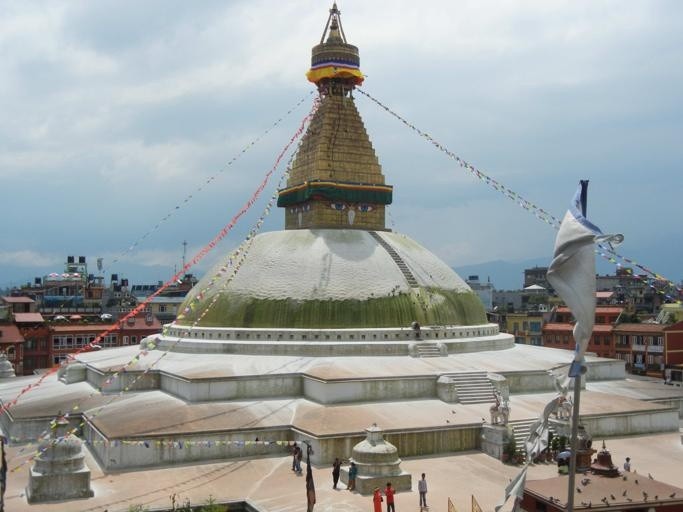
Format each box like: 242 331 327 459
542 181 623 377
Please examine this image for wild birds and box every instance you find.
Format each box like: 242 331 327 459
576 473 678 509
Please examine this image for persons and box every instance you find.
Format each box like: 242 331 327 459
418 473 429 512
294 447 302 475
332 457 349 489
373 487 383 512
623 457 631 473
557 459 568 475
345 462 357 491
292 443 298 470
384 483 395 512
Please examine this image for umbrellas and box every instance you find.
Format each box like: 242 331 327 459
99 314 112 319
557 451 571 459
48 272 81 280
53 315 65 321
69 314 81 320
